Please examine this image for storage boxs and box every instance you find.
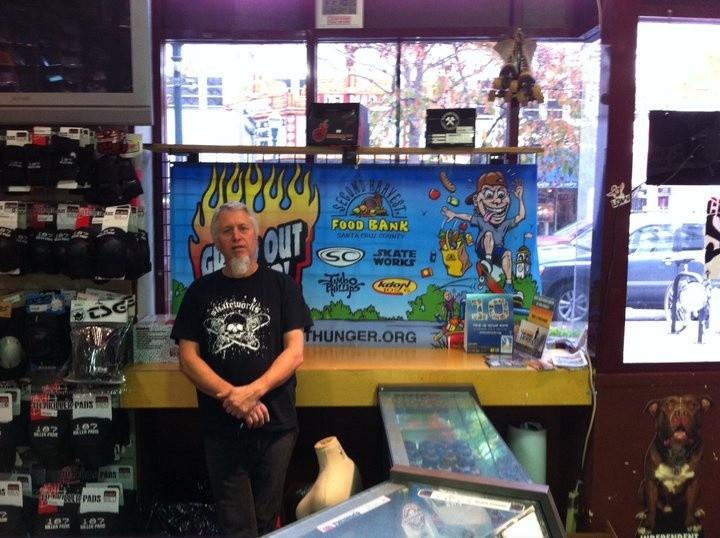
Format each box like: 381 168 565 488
306 101 369 145
427 109 475 147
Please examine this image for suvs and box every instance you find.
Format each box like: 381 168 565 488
535 211 706 325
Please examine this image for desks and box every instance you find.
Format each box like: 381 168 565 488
121 346 597 537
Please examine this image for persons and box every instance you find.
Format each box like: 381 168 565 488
170 202 315 538
